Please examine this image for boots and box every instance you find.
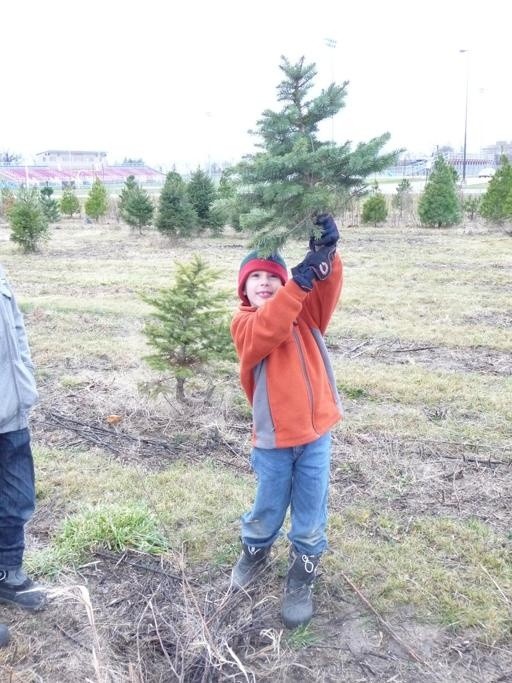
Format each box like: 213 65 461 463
223 536 278 591
281 544 322 629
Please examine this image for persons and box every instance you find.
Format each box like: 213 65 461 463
0 267 46 649
230 210 345 629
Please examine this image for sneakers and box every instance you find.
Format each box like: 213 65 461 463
0 569 44 608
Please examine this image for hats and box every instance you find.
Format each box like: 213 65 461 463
238 249 288 306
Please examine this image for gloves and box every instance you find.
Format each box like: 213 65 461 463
291 246 336 291
309 214 339 257
